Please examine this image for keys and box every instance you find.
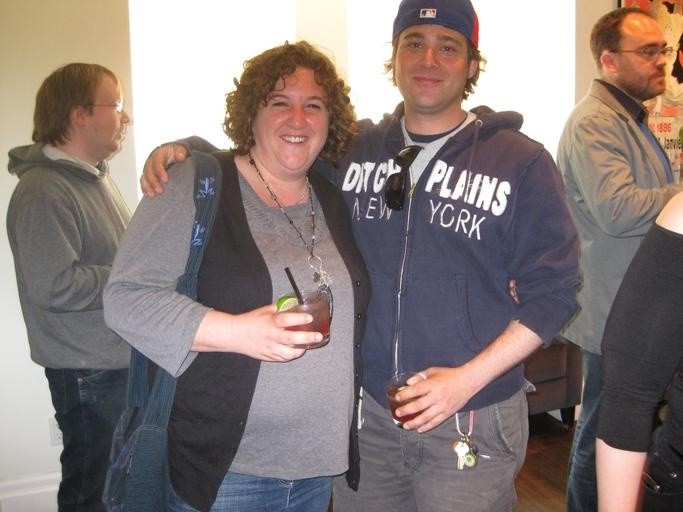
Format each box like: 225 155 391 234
452 442 470 470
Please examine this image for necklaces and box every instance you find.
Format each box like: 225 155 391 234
245 149 332 289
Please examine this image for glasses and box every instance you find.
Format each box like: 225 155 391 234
615 45 673 58
91 100 123 113
384 145 422 209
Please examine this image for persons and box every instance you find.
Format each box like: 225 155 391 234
564 178 680 511
100 37 371 511
3 62 137 511
136 2 586 511
547 7 681 479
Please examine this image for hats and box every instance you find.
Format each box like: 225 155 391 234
392 0 480 48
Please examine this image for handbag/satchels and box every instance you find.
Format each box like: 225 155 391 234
101 410 174 511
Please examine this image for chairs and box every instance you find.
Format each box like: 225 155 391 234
525 342 582 430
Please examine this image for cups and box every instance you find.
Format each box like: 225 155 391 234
276 283 335 350
387 370 427 427
